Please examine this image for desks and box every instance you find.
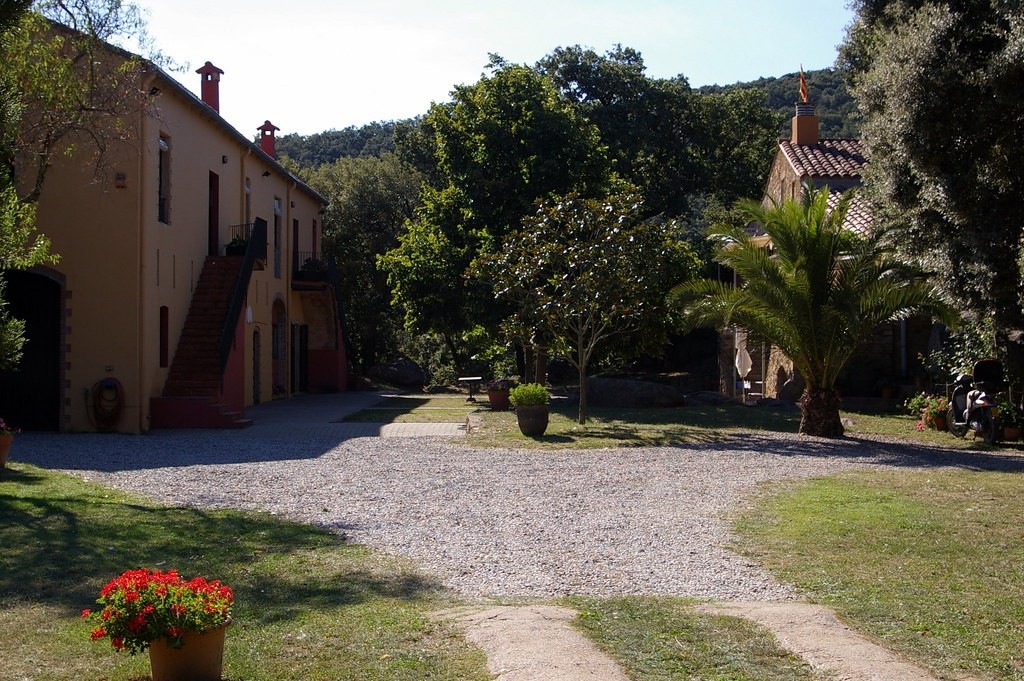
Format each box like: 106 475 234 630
458 377 482 402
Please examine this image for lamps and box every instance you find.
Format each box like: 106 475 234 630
319 210 324 215
152 87 162 98
262 170 271 178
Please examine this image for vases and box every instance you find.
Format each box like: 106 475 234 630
488 391 511 412
0 435 12 467
147 615 232 681
934 415 946 430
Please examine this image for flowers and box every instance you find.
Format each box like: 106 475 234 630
80 567 235 656
487 378 516 391
0 417 22 437
904 390 949 432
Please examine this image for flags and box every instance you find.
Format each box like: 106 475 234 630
798 69 807 102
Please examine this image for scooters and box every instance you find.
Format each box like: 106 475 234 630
946 357 1005 445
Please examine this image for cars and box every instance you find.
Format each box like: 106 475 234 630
684 391 730 408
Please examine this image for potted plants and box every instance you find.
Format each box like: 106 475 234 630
223 235 250 257
508 382 551 437
996 401 1019 440
